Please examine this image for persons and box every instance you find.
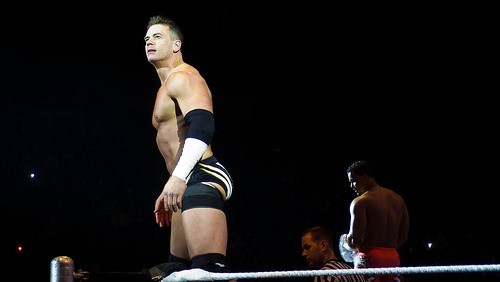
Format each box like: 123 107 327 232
342 158 411 282
140 13 234 279
296 223 366 281
